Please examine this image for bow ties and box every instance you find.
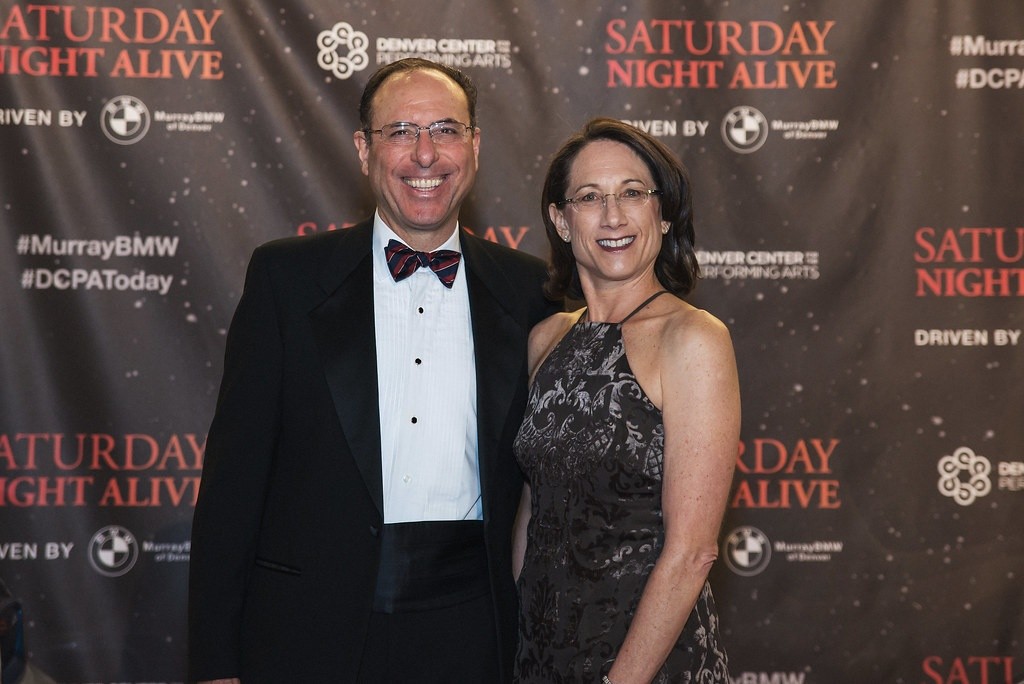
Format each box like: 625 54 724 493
384 239 461 290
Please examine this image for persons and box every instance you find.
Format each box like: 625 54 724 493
190 58 565 684
511 119 741 684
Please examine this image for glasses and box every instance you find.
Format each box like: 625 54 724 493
555 187 664 213
365 122 474 145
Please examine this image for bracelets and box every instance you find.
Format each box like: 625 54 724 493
602 675 612 684
